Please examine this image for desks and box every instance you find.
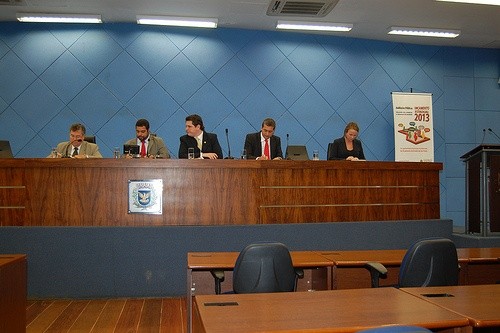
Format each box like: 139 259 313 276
187 246 500 333
0 158 444 228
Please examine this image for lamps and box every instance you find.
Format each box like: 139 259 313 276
15 13 102 23
137 15 218 29
386 26 460 38
276 20 353 32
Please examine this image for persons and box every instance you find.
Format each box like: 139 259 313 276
46 123 103 158
178 115 223 159
124 119 167 159
243 118 283 160
330 122 366 161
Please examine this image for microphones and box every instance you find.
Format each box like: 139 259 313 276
148 139 152 154
482 129 485 144
224 129 234 159
62 139 82 158
488 128 500 139
285 134 288 160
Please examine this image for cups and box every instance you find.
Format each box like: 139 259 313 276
51 148 59 158
113 146 120 159
188 147 195 159
312 150 319 160
241 149 246 159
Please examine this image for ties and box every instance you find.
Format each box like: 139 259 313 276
141 140 146 156
72 147 78 156
264 139 271 159
196 136 203 151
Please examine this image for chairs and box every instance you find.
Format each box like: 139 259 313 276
285 145 309 161
366 237 463 288
210 242 304 296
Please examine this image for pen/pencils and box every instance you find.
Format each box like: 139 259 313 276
264 153 265 156
